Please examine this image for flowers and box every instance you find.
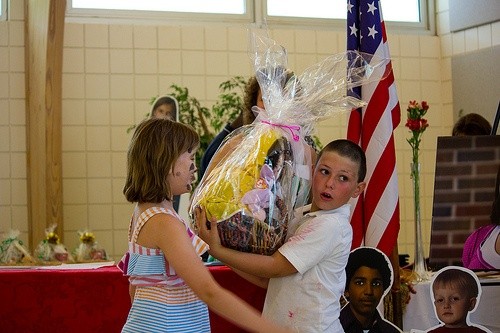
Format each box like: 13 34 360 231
405 100 430 262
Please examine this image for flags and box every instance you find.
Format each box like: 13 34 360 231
345 0 402 258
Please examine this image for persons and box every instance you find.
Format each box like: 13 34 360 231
462 173 500 270
193 138 367 333
427 269 488 333
198 64 304 178
117 118 295 333
150 96 181 214
338 247 402 333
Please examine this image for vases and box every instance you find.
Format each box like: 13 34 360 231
414 211 433 282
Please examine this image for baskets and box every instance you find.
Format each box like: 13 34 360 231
189 130 295 254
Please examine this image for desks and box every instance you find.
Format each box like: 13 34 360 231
0 261 267 333
